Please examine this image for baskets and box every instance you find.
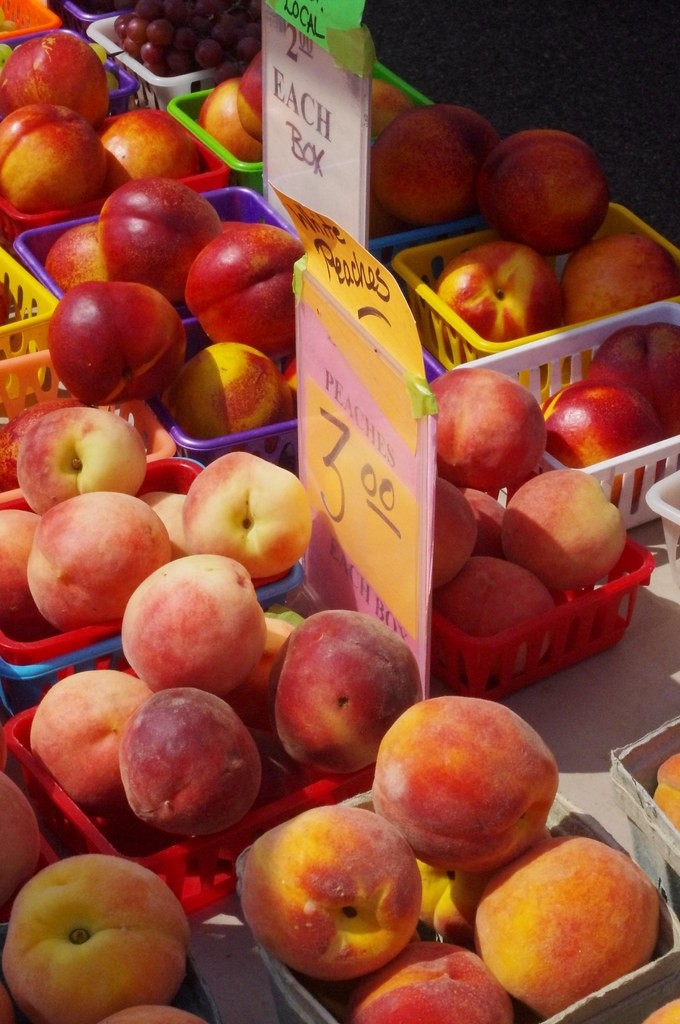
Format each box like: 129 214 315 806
0 0 679 1024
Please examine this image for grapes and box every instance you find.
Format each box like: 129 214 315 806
0 0 264 92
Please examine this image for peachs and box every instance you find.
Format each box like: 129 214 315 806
0 29 680 1024
